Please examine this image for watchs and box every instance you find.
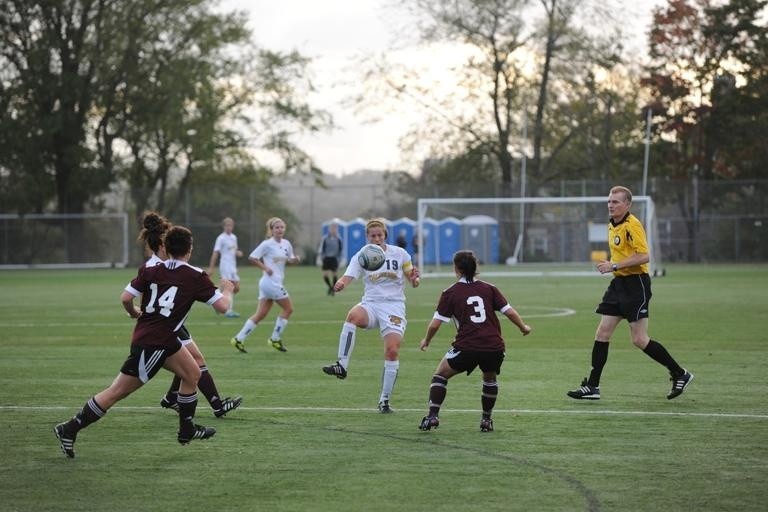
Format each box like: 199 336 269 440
611 264 618 271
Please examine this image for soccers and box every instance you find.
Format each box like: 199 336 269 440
358 244 385 270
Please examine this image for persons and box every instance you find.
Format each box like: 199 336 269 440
52 225 216 457
397 229 407 249
412 227 425 267
419 250 530 431
324 218 421 415
231 217 298 352
140 212 241 417
207 216 244 317
320 222 343 295
566 185 694 400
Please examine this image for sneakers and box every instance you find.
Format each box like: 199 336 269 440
418 415 439 431
323 361 347 380
269 339 286 353
480 419 494 431
231 338 246 355
667 368 694 399
53 391 243 458
567 376 601 400
378 399 392 413
225 311 239 316
